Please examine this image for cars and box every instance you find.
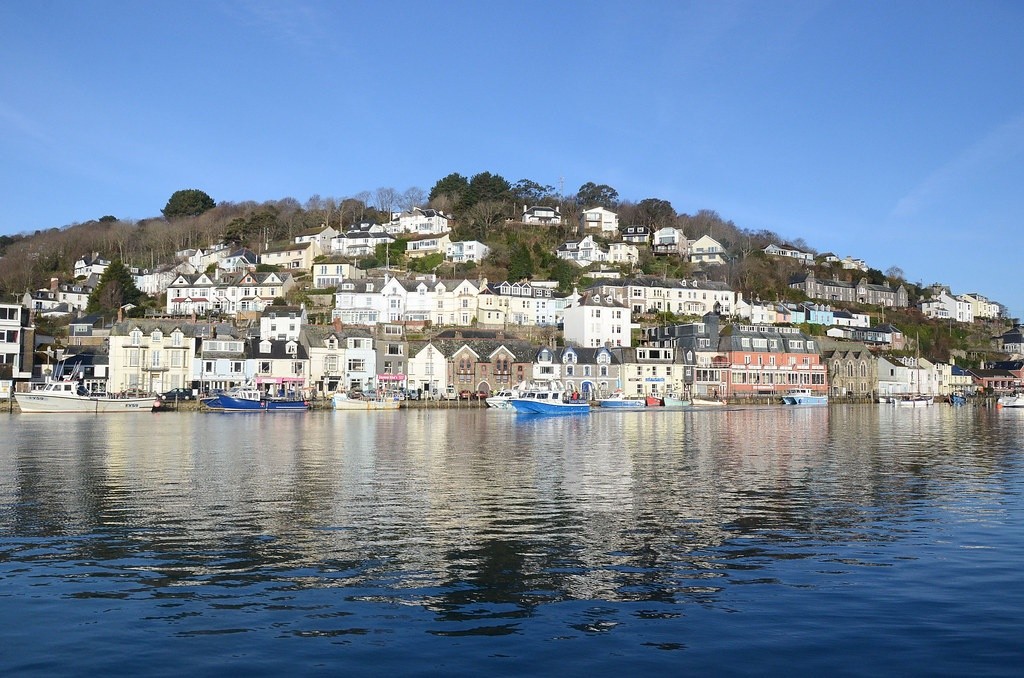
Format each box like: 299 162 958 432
205 388 225 396
460 390 472 400
157 387 193 400
432 388 456 400
117 387 148 399
92 388 106 396
404 390 418 401
361 390 377 397
473 391 487 400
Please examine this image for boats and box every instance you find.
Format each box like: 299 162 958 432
595 391 646 406
331 391 401 410
782 388 829 403
691 398 726 405
508 388 591 414
486 390 518 408
646 395 663 405
663 398 689 405
14 382 159 413
200 375 312 411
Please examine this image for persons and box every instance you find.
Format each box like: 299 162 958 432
713 389 718 398
311 386 318 401
381 384 422 401
573 391 578 401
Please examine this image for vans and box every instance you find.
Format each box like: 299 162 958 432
223 386 255 397
